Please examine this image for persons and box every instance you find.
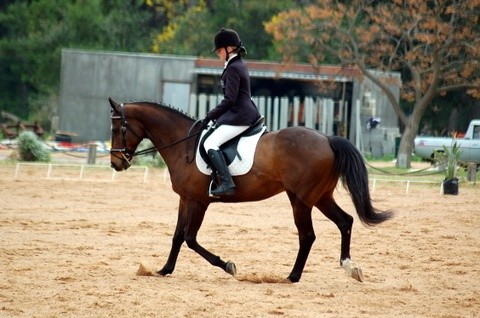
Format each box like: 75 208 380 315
202 29 255 194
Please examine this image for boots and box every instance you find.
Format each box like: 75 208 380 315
207 149 236 194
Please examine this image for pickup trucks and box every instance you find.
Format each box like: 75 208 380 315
413 119 480 168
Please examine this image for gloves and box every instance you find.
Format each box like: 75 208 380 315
202 116 211 129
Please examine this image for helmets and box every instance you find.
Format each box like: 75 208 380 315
212 28 241 52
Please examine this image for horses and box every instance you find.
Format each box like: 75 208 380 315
107 93 395 284
